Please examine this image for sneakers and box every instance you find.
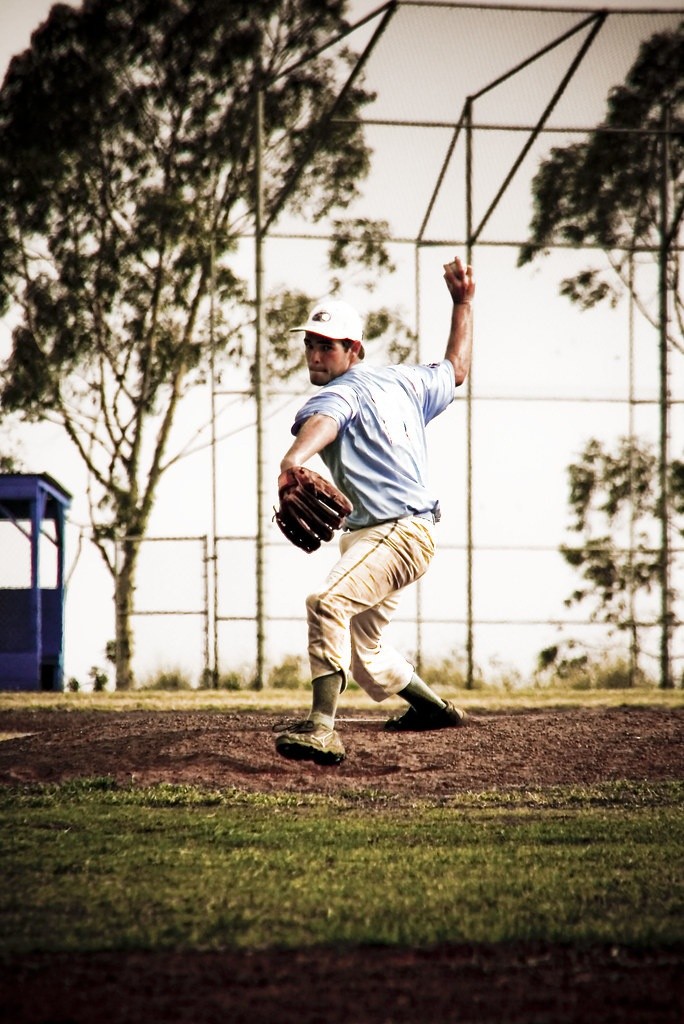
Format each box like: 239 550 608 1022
385 698 468 731
272 720 346 765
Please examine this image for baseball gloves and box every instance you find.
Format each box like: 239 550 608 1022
272 466 353 555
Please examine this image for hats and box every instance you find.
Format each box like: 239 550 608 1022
289 301 363 344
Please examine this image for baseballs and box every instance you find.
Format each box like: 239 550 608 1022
449 258 468 276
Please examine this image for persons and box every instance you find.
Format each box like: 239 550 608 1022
276 256 475 765
